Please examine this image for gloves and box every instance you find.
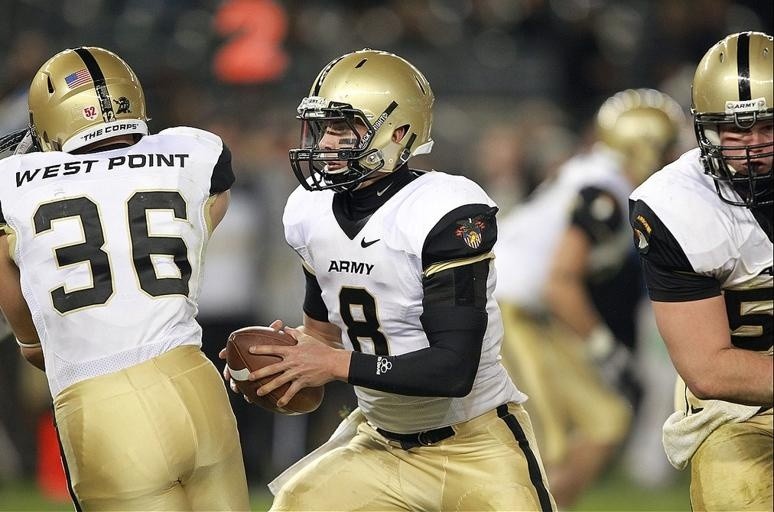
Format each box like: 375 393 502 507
1 127 34 155
585 325 641 396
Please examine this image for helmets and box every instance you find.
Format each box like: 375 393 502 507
286 47 436 192
600 87 688 185
26 45 149 154
691 30 774 209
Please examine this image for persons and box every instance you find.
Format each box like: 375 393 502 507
492 93 688 510
629 32 774 511
0 46 250 512
218 51 557 512
0 1 772 494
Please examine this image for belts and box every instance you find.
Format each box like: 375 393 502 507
376 427 455 450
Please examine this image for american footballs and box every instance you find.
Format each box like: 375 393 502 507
227 326 325 415
